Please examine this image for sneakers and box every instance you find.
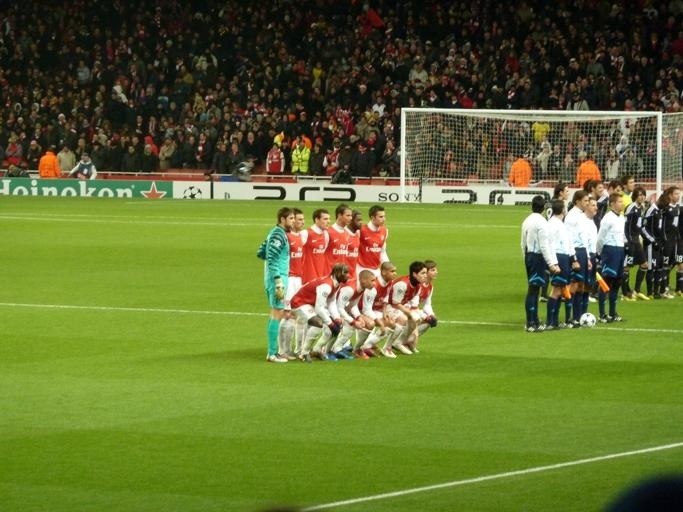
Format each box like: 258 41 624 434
672 289 683 297
544 324 560 330
352 347 369 359
380 347 397 358
525 323 539 332
535 322 544 332
571 319 580 327
632 289 651 301
309 351 329 361
564 321 579 328
363 347 377 357
322 352 337 361
542 322 550 330
402 340 419 353
598 314 613 323
277 352 297 360
334 350 354 359
608 313 624 322
293 350 301 355
298 353 313 363
286 351 299 358
391 340 413 354
343 345 353 352
621 294 637 302
645 291 661 298
659 292 674 299
265 353 288 363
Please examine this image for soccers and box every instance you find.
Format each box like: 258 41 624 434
580 313 596 327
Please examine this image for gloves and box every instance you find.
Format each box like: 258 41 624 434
596 253 601 260
275 286 285 300
423 316 434 325
328 322 340 337
429 314 437 328
652 241 660 252
624 242 630 255
256 240 266 260
334 319 343 330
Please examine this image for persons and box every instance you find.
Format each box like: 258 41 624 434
635 194 674 301
380 261 427 359
285 207 303 355
663 186 683 298
299 208 331 286
577 159 600 188
582 196 598 315
553 1 577 182
620 176 635 215
577 0 600 161
395 258 439 354
256 207 296 363
597 180 622 230
520 196 556 333
563 190 592 326
539 182 570 302
597 192 626 324
353 262 396 361
312 269 377 361
356 204 389 292
290 262 349 363
544 200 576 330
587 181 604 304
620 187 651 303
327 203 352 273
601 0 683 177
